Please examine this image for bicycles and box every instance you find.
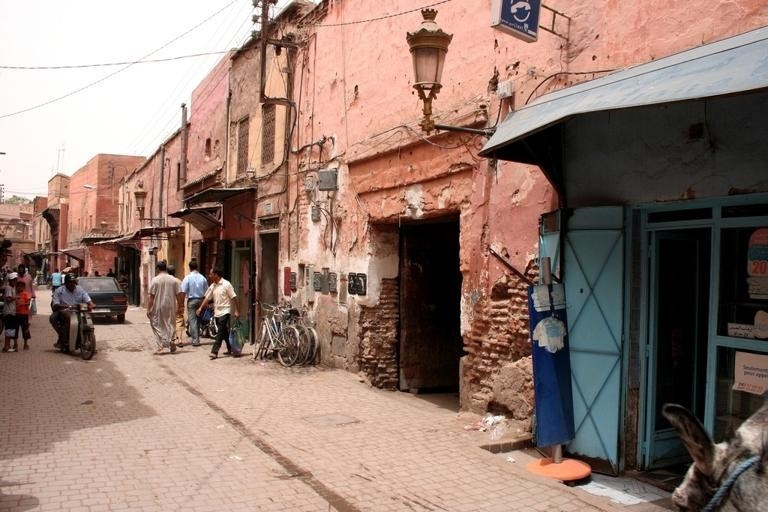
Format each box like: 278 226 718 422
250 296 320 369
184 300 219 340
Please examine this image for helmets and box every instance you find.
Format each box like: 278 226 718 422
65 272 77 284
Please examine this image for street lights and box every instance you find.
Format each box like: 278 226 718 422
0 152 7 155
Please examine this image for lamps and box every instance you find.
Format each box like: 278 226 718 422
406 8 495 135
134 183 164 222
100 220 118 236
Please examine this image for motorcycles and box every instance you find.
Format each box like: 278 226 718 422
56 303 98 361
0 275 5 338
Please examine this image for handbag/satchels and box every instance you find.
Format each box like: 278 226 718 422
228 318 245 354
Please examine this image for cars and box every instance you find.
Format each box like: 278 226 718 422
74 275 129 324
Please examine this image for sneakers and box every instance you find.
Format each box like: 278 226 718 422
169 337 200 353
208 350 231 360
2 342 31 353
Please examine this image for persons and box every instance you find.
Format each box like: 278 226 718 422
0 263 130 352
167 265 184 347
180 261 209 346
147 261 183 355
196 267 240 358
